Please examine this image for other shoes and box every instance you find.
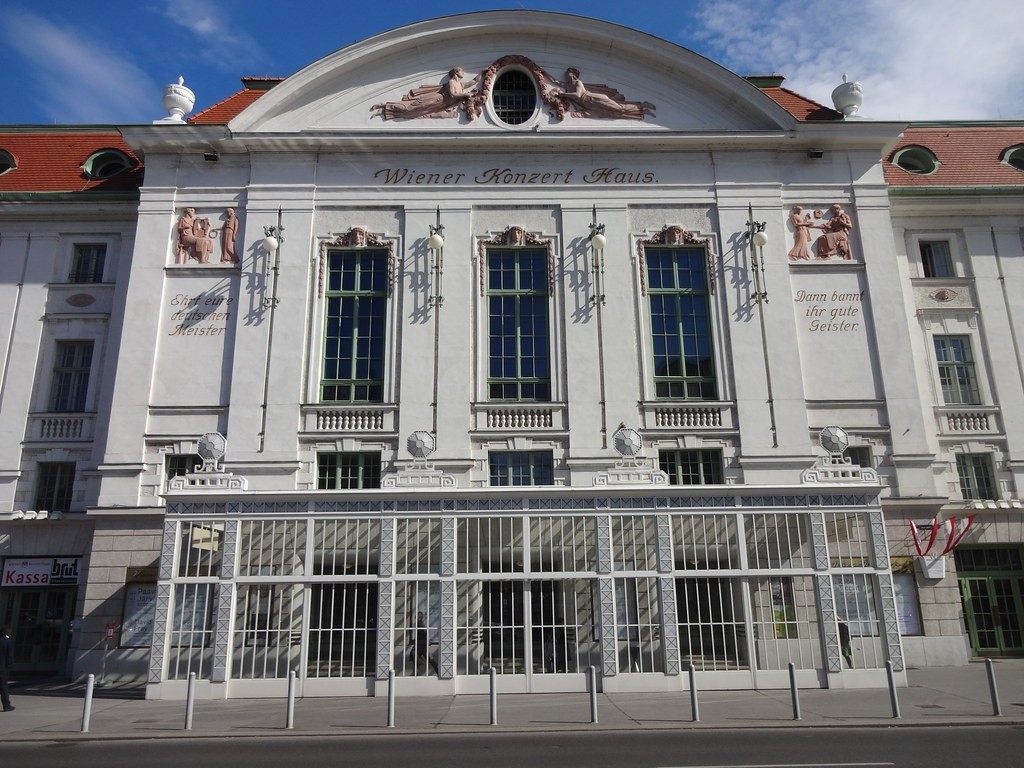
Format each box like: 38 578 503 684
4 706 16 712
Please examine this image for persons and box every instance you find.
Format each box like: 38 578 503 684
1 626 15 713
344 227 367 247
788 205 853 260
664 225 683 245
502 226 530 246
369 65 491 121
836 616 855 670
178 208 241 263
539 66 657 120
